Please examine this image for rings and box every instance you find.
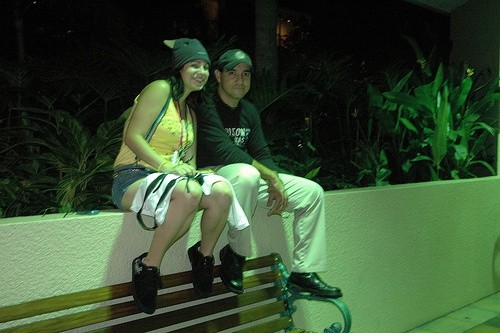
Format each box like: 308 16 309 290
284 206 287 207
281 205 284 207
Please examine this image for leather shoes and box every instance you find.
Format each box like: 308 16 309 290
220 243 246 294
286 271 343 298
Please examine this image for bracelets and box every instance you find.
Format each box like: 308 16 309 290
158 161 171 172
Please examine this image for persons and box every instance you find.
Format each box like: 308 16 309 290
195 49 344 298
113 39 233 315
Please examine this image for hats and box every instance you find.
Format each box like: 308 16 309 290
216 49 253 71
170 38 211 70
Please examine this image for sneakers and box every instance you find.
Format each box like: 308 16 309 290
187 241 216 298
131 252 160 314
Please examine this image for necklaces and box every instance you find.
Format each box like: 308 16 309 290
178 100 195 156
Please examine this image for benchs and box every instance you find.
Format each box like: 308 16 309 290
0 253 352 333
